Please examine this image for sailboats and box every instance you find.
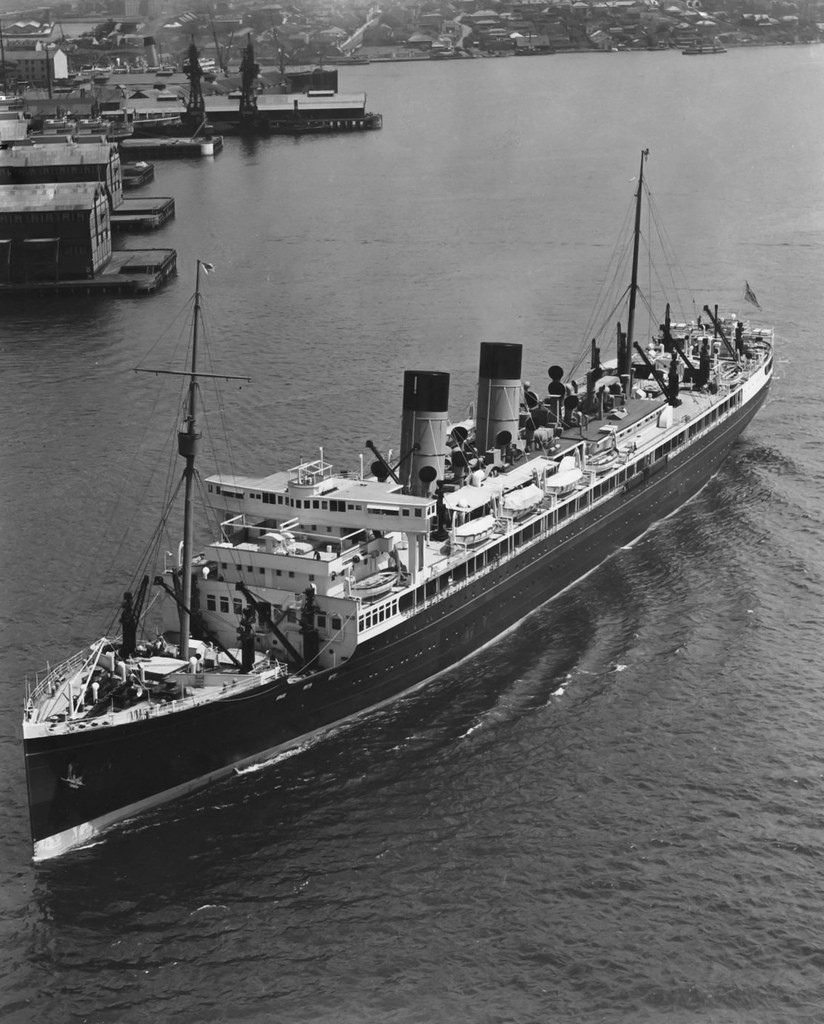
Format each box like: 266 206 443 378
0 5 384 137
20 148 780 866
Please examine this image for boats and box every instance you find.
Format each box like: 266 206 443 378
20 109 223 157
1 183 178 294
1 144 177 228
